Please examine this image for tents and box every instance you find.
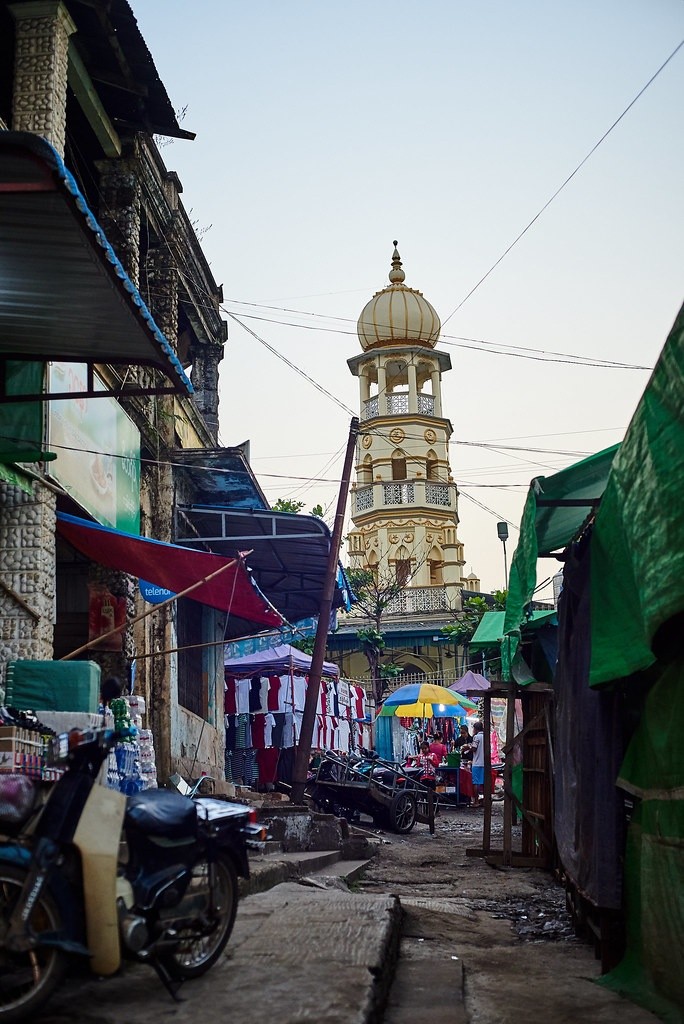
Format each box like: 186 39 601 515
224 643 339 678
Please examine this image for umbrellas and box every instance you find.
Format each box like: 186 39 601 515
375 682 481 759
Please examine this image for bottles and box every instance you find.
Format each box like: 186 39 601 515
98 694 158 796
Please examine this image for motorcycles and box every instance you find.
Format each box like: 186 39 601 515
309 744 425 822
0 716 266 1023
458 750 506 802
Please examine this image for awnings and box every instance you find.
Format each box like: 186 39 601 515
56 514 310 636
0 126 196 397
175 439 358 634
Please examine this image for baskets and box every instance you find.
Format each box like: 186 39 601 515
0 772 44 841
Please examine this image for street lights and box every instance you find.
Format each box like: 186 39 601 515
496 521 512 686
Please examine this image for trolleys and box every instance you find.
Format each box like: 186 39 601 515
316 755 456 833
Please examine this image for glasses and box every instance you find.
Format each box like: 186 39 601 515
460 731 467 734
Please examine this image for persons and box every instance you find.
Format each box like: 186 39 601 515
407 719 485 833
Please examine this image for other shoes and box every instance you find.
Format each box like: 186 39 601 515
470 804 481 808
